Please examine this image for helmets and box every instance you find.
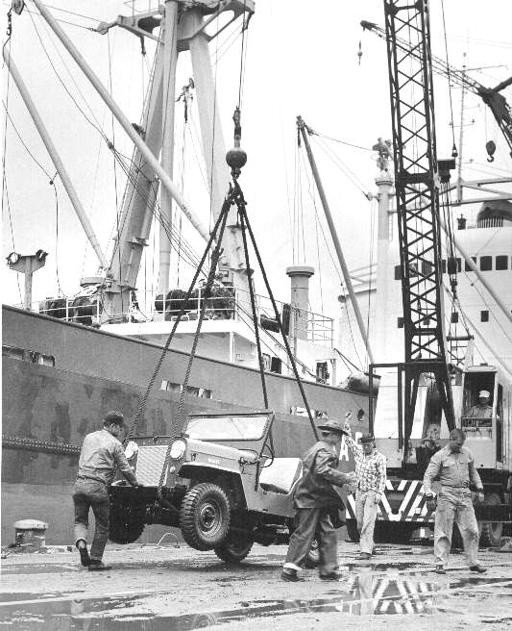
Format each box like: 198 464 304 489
317 420 349 435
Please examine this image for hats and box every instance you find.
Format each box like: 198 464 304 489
479 389 491 398
360 433 375 443
104 410 126 426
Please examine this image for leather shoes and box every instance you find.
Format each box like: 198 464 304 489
360 552 370 559
89 562 112 570
280 571 305 582
470 564 487 572
77 540 90 566
320 573 350 581
436 564 446 573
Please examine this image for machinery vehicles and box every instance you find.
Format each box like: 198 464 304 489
351 0 511 551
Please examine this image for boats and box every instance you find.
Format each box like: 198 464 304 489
2 0 335 414
2 123 510 629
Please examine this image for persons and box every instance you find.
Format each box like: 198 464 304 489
421 428 489 573
463 388 499 428
110 425 140 482
353 433 388 559
280 417 356 583
70 410 144 570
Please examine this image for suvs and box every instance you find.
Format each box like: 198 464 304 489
107 405 322 569
